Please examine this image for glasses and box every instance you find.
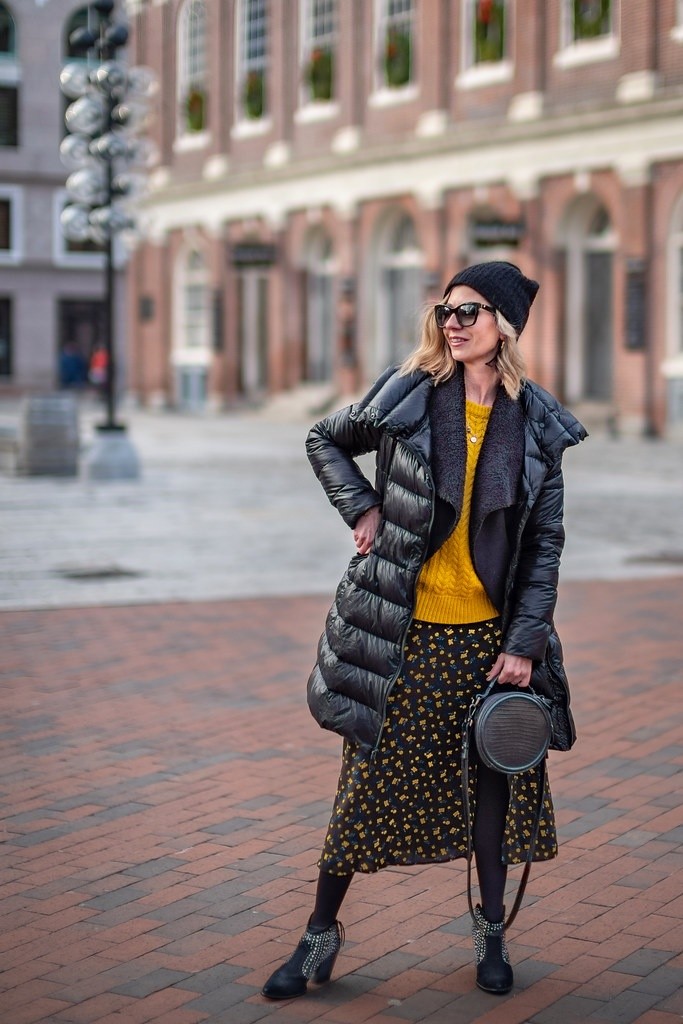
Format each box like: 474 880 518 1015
434 301 493 328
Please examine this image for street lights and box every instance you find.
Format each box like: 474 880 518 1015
57 0 161 431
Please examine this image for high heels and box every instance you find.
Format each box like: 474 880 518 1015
261 911 347 1000
471 904 514 995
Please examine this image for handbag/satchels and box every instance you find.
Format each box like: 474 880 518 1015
475 676 554 774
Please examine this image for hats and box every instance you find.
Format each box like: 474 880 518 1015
443 261 540 342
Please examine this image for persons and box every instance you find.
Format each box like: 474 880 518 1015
256 260 590 1001
53 342 107 404
